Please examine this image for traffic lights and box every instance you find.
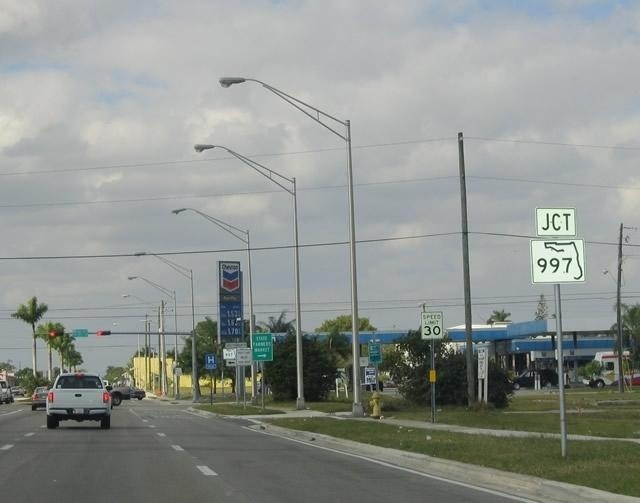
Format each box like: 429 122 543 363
49 330 65 337
97 330 111 337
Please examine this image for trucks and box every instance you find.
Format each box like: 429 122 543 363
581 349 640 389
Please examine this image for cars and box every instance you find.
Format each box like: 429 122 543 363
30 386 48 411
102 378 147 408
509 367 557 391
383 378 398 388
10 386 27 396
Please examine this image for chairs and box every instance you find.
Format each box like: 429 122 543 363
63 381 97 388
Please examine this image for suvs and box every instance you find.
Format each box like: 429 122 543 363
0 380 15 403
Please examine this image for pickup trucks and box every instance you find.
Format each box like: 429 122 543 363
44 372 114 430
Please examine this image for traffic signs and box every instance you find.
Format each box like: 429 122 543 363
251 332 274 362
422 312 443 339
72 329 89 337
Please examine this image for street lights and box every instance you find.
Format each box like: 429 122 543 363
216 74 367 420
112 248 203 404
601 269 626 395
171 207 262 407
193 143 306 412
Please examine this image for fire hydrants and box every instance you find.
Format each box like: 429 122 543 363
368 389 384 418
